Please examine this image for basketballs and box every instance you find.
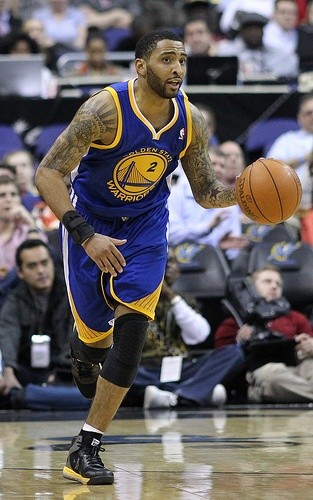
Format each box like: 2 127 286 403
235 159 302 225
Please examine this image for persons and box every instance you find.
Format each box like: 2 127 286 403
34 30 264 485
0 239 94 412
0 120 85 297
163 89 313 264
120 247 243 408
0 0 313 77
215 266 313 403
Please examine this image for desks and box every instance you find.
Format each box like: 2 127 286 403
55 82 296 145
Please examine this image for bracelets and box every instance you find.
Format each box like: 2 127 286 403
62 210 95 245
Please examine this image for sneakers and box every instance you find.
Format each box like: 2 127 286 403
145 409 177 433
210 384 227 406
63 435 114 485
213 409 227 430
143 386 178 410
70 322 102 400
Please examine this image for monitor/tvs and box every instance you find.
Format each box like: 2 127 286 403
186 55 237 85
0 54 46 98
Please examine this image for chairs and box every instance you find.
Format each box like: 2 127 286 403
158 222 313 357
